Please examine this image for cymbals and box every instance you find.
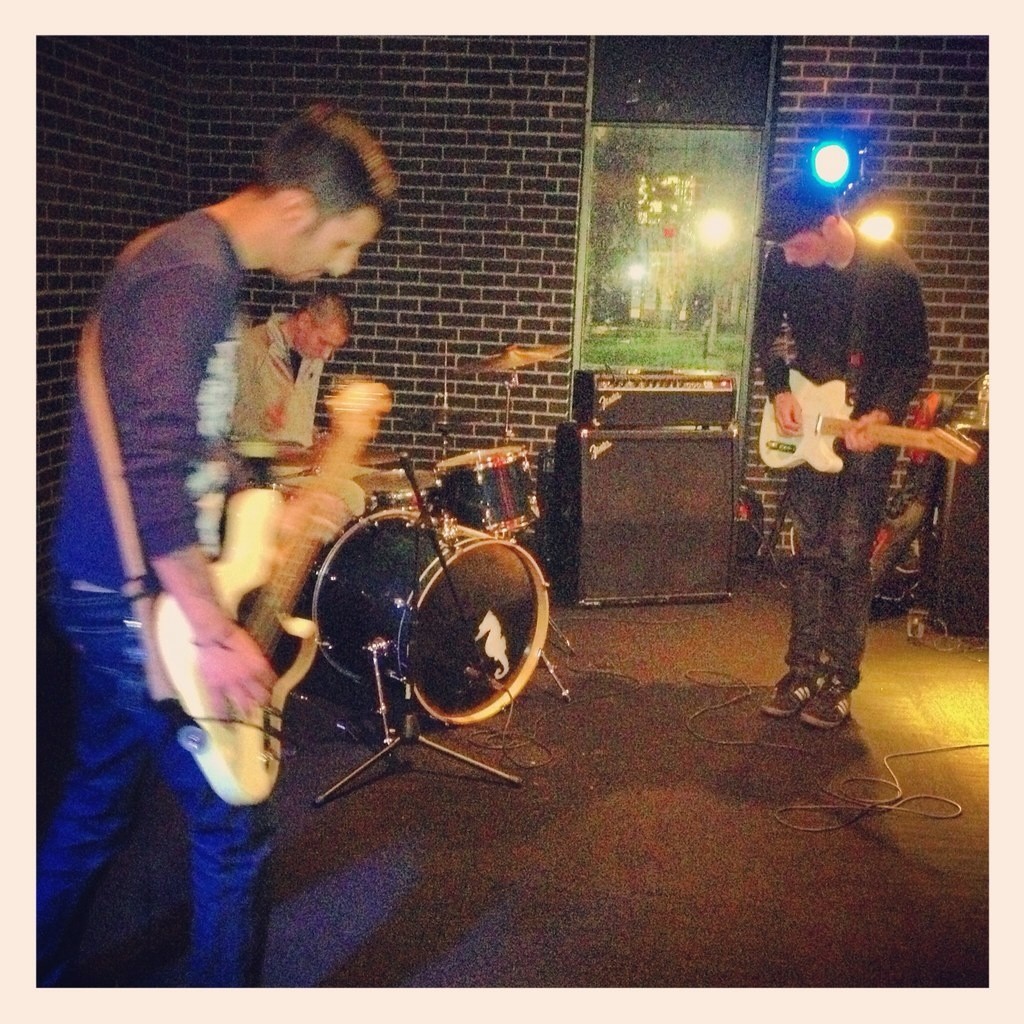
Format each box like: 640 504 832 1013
225 434 307 459
460 343 576 377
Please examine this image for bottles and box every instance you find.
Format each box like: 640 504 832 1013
978 375 989 427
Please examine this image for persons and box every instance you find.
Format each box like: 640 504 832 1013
227 293 353 489
37 109 402 988
755 176 934 732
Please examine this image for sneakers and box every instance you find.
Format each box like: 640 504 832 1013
802 676 851 728
761 667 818 717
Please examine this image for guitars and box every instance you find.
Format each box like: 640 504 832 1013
152 381 395 805
758 366 983 473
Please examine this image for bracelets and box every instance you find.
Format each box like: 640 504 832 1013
191 627 235 652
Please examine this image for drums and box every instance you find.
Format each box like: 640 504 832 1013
437 445 544 538
301 504 551 728
354 468 440 511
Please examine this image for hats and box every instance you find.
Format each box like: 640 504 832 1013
754 172 838 241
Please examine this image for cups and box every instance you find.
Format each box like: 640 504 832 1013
905 607 929 647
